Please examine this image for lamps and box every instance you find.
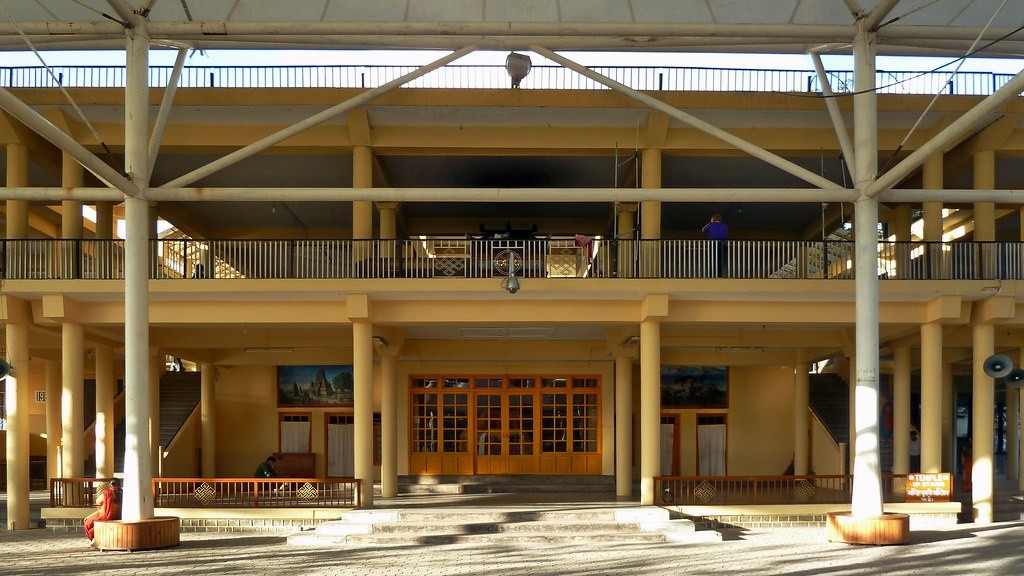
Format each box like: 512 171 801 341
505 53 531 88
506 273 520 294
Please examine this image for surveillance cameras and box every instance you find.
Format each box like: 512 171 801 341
506 277 520 294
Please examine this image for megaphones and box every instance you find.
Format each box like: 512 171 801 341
1006 368 1024 389
982 353 1014 378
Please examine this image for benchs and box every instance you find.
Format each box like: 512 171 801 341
827 511 910 545
95 515 180 551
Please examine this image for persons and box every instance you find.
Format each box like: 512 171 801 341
879 400 893 442
256 457 277 478
961 430 972 491
702 213 728 278
419 410 436 452
910 431 920 473
193 264 207 278
84 478 123 545
